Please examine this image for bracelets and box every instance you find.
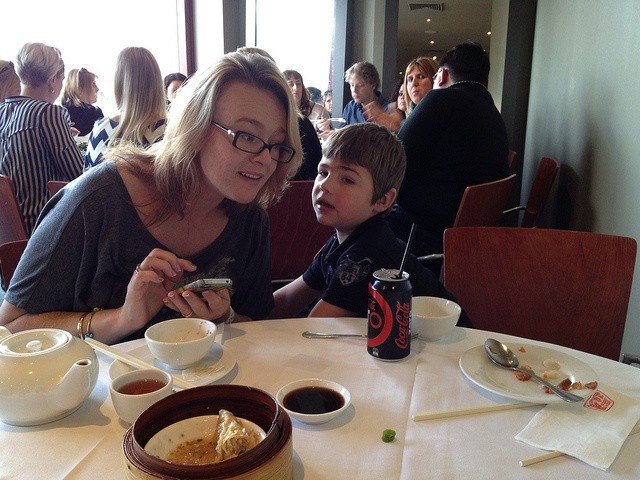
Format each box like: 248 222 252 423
84 306 103 339
76 311 90 340
225 305 235 323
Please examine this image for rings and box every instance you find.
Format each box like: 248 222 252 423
136 264 141 272
181 311 193 318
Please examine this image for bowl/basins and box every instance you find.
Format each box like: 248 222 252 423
412 296 462 341
144 318 217 370
123 382 291 479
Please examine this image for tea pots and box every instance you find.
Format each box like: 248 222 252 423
1 326 99 427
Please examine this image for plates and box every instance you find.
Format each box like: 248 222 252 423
459 343 599 404
109 343 236 389
277 378 352 424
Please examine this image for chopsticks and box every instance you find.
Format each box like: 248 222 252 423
310 118 346 123
411 404 546 422
82 336 195 389
519 427 640 467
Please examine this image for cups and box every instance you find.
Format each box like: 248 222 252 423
108 369 173 424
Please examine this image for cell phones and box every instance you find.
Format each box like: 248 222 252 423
177 278 233 297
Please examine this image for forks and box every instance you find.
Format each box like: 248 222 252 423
303 331 420 341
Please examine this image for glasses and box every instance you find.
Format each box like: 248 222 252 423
432 67 443 82
77 68 88 74
210 121 296 164
0 60 14 78
51 45 61 61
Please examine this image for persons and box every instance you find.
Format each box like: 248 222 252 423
163 72 188 108
272 121 476 329
61 66 104 137
323 90 332 118
383 40 510 280
0 60 81 137
236 46 322 181
306 86 323 104
314 62 390 143
84 46 167 173
388 77 407 119
0 42 84 240
281 69 330 124
0 51 304 345
363 56 439 136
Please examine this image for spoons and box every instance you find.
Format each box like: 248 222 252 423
485 338 583 403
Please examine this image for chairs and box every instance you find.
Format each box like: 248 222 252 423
521 156 560 228
265 180 337 280
507 150 519 171
443 226 638 362
453 173 517 228
47 180 70 197
0 175 28 243
0 239 28 292
